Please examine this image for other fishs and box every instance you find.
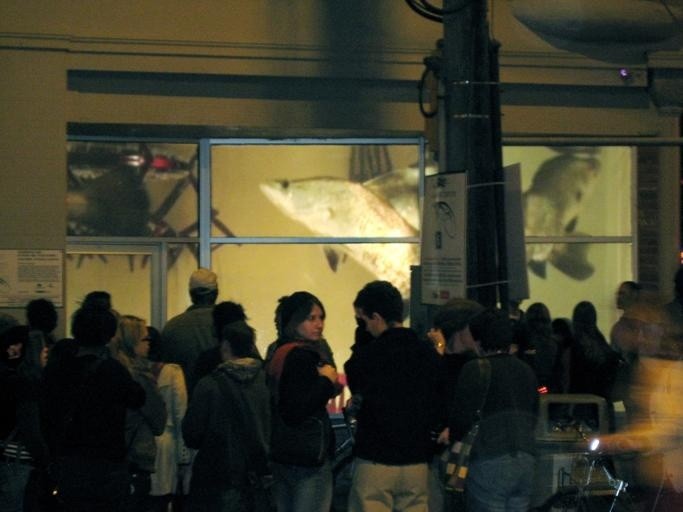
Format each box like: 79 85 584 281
66 135 199 241
258 153 605 302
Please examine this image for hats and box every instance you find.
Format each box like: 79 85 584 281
70 291 121 320
188 269 218 295
439 297 490 329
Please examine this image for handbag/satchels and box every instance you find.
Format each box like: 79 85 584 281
438 425 478 494
176 428 193 465
269 415 331 468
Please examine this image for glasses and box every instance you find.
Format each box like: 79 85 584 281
355 315 368 328
141 336 152 341
